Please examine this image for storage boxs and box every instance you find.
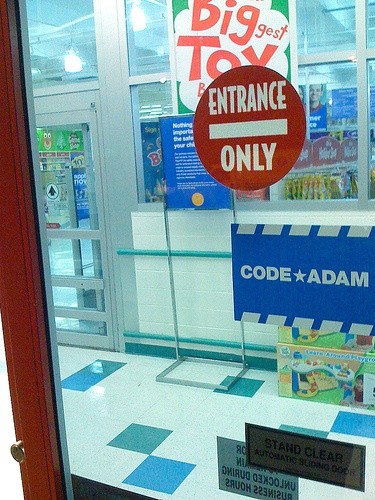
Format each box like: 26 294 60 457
277 325 375 354
276 342 375 411
